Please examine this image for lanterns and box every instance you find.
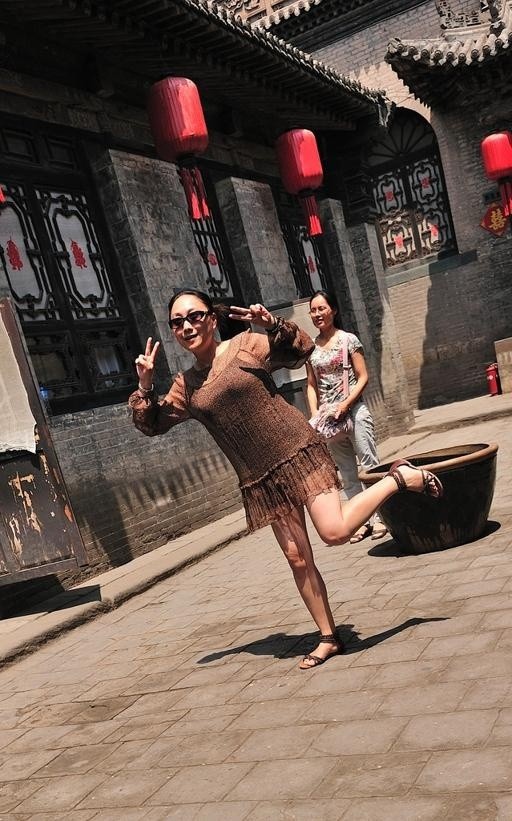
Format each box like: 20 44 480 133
481 129 512 216
275 127 325 237
149 75 211 221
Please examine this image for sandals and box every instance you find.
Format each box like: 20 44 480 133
347 524 387 544
299 628 346 669
383 458 444 499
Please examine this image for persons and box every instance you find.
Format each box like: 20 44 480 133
126 288 442 670
305 288 384 544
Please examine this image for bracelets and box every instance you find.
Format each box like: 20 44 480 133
138 382 154 395
265 315 281 333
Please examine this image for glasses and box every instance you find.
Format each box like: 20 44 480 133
167 309 213 329
307 307 331 316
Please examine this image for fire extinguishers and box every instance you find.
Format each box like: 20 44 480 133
485 363 498 395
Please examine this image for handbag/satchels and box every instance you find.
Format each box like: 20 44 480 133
316 403 354 443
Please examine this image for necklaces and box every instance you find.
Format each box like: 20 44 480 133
197 353 214 368
